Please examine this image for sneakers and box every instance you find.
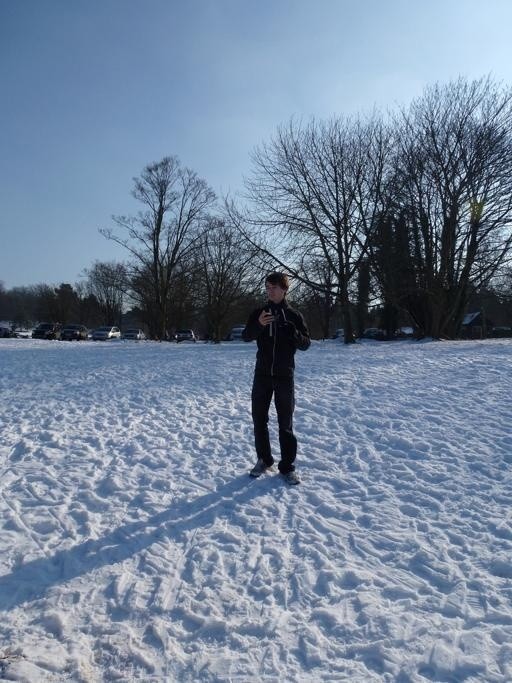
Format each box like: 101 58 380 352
282 469 301 485
250 456 274 478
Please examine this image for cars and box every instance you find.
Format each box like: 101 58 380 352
0 317 246 342
332 323 418 341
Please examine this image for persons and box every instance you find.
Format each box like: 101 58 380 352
239 269 310 489
